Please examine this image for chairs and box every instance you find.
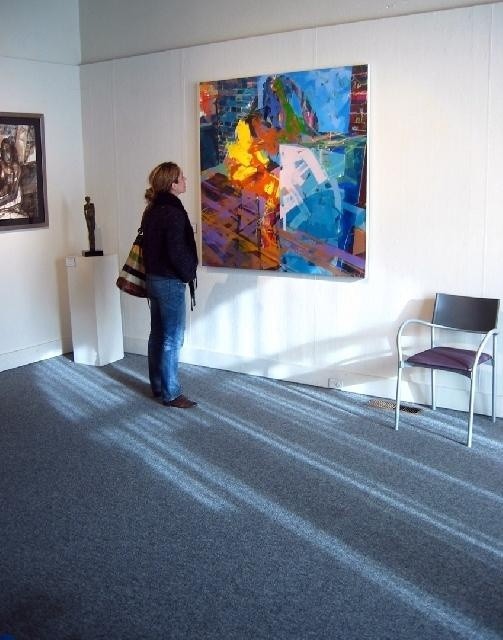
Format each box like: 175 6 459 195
394 294 500 448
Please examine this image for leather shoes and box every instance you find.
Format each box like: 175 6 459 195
163 393 198 408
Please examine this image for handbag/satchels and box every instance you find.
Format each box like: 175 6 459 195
115 227 150 299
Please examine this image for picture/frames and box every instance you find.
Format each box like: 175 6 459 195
0 112 49 232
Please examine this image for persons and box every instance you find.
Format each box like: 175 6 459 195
139 161 197 409
83 196 96 251
